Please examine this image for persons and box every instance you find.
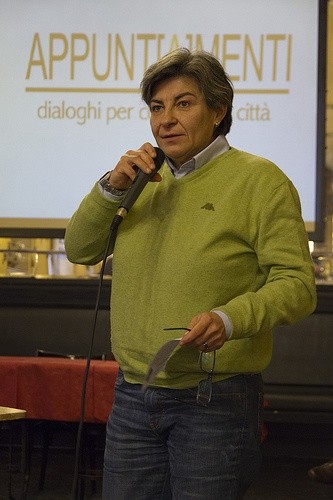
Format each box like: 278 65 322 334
66 49 317 500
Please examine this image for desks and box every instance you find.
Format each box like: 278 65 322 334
0 355 120 500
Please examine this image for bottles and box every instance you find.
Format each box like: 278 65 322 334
48 239 74 277
311 249 333 284
6 237 39 277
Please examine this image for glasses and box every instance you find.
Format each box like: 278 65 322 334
157 328 215 408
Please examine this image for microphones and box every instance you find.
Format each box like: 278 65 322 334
109 147 165 232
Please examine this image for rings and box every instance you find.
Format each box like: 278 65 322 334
204 342 208 349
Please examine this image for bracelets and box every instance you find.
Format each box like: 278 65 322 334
99 170 125 196
